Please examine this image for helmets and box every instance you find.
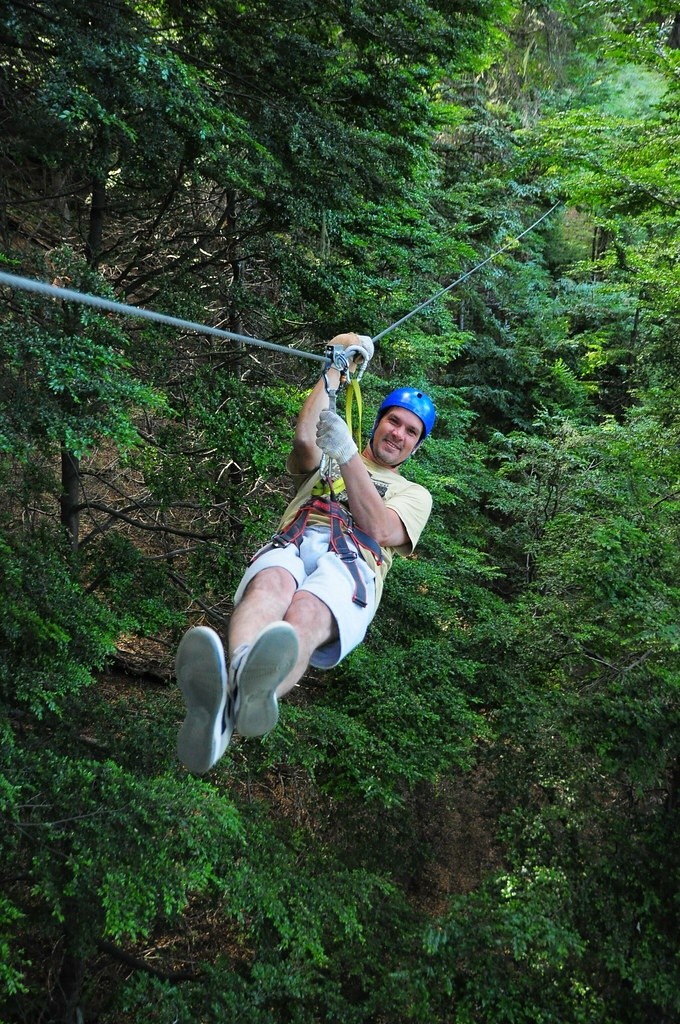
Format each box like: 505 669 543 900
375 387 435 440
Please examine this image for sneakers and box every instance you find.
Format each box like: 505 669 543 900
175 626 235 774
228 620 300 737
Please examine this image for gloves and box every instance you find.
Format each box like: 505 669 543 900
325 331 375 371
315 408 359 465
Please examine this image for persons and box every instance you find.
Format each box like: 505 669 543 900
175 334 434 775
